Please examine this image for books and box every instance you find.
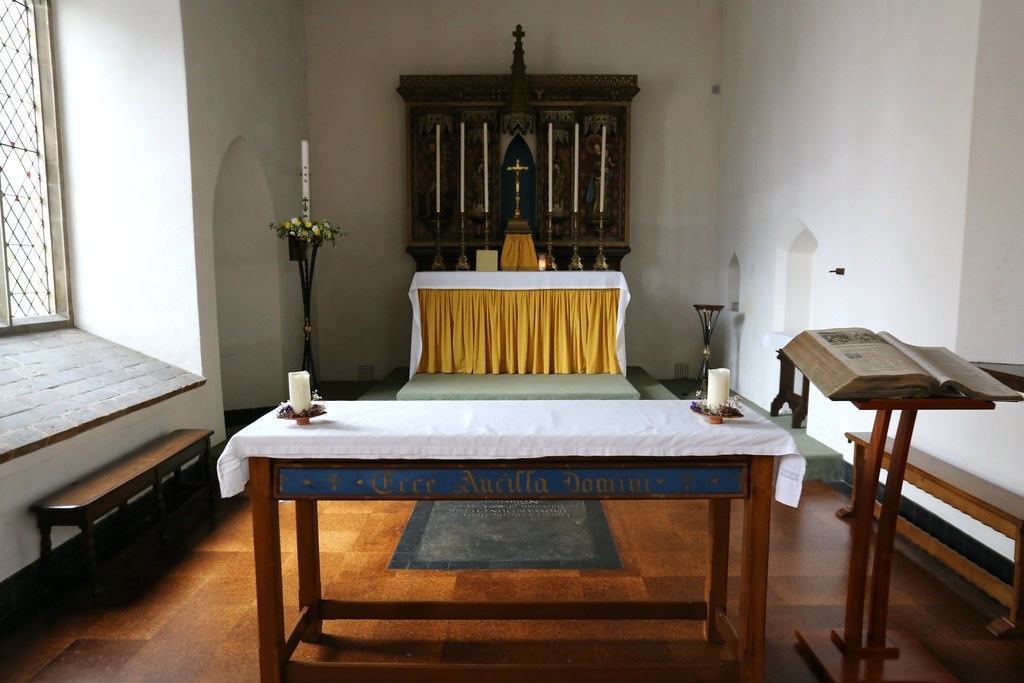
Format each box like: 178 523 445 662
782 328 1023 401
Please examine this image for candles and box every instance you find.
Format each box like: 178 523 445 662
548 122 552 213
484 122 489 214
706 368 730 414
300 140 310 223
460 122 464 214
573 123 579 213
600 125 606 213
436 124 440 212
287 369 310 411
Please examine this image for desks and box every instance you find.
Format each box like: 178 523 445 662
217 399 808 683
693 303 725 390
408 270 631 382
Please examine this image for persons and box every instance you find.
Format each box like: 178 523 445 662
423 137 615 233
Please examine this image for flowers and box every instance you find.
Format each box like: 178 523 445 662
265 214 345 249
276 388 326 418
689 390 742 416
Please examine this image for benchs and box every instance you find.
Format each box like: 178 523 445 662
28 427 216 600
835 429 1024 641
